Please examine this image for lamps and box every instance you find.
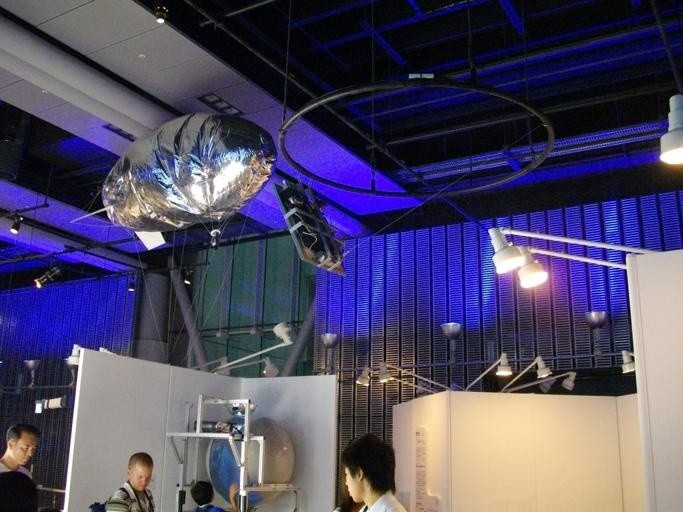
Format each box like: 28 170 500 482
487 225 649 290
34 255 61 290
67 320 294 378
10 210 24 234
660 93 683 166
355 352 579 392
621 350 634 374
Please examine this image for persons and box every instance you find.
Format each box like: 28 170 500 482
337 432 407 512
102 450 155 512
189 480 228 512
0 422 41 511
